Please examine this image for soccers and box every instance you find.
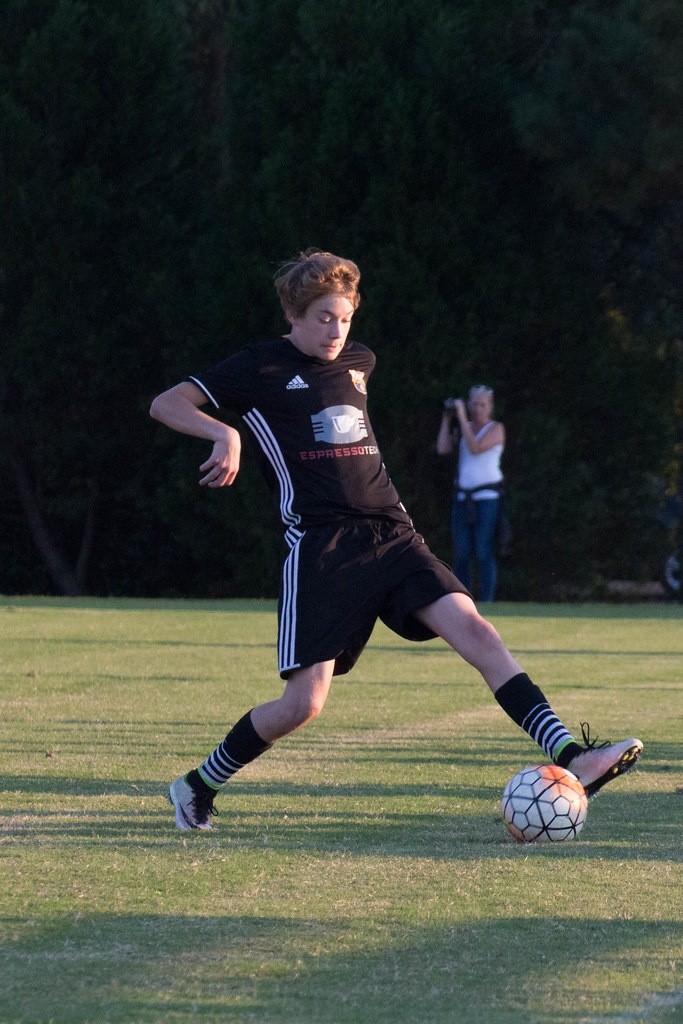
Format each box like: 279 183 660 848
502 764 585 841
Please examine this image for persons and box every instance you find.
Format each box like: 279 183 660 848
149 252 645 832
436 386 506 603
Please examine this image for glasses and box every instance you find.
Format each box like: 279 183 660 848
471 385 494 394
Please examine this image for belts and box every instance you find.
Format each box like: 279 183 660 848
455 489 499 501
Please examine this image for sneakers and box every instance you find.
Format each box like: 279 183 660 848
664 556 681 591
169 770 219 832
566 722 644 798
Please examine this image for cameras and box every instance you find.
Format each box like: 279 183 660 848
445 399 456 413
